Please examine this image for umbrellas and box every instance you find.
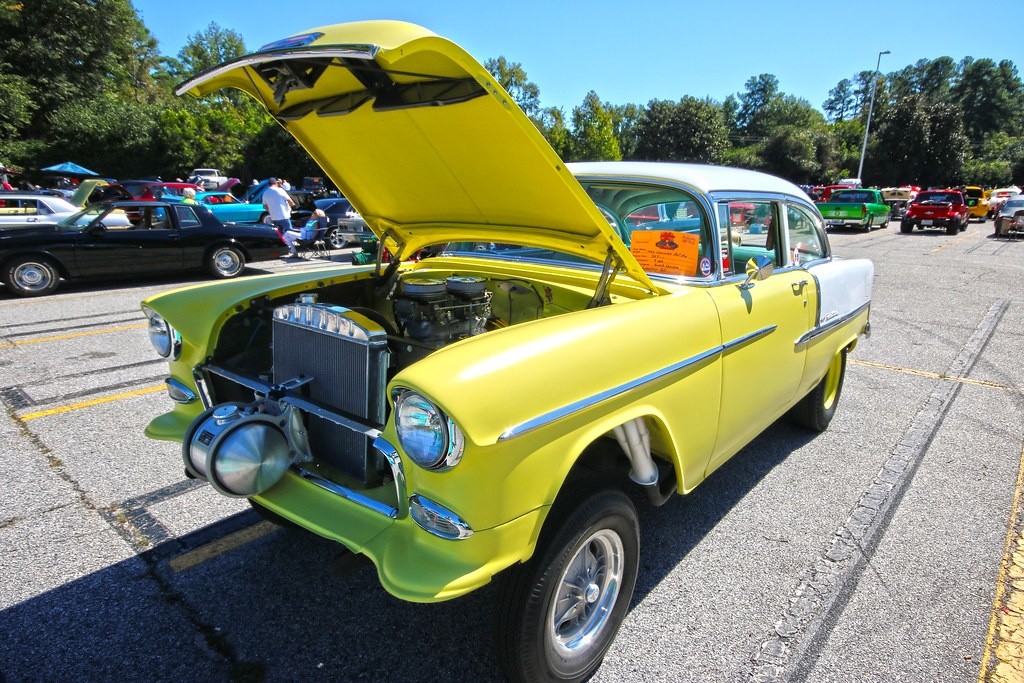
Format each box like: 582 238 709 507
40 161 100 176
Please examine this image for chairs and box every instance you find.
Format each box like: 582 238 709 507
297 215 331 262
137 219 198 231
351 235 421 265
997 209 1024 241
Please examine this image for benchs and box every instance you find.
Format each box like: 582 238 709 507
698 244 777 273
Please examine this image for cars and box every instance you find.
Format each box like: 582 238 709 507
140 19 877 682
994 195 1024 239
0 168 524 259
627 176 1024 223
0 197 291 298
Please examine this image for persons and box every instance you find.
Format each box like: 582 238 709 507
1 179 19 191
892 200 906 216
943 185 968 206
179 187 199 205
281 208 326 259
71 177 78 185
155 176 162 183
261 178 295 234
200 180 205 191
6 166 16 173
743 211 754 232
807 185 818 200
277 178 291 191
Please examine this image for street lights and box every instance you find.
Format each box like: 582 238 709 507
857 50 893 180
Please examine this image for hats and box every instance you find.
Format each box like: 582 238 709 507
72 178 79 185
269 177 277 184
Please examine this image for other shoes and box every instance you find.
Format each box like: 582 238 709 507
287 252 299 258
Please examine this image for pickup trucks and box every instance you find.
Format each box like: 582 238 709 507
814 188 893 232
901 186 970 236
